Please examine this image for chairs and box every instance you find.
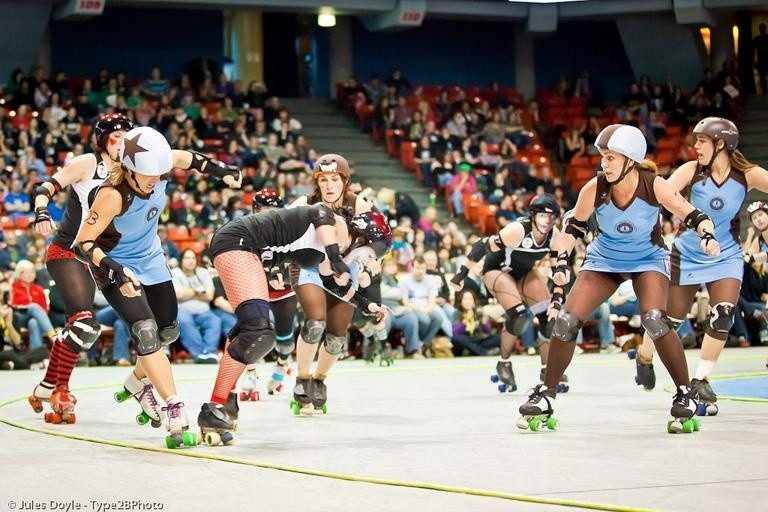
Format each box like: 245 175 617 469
334 80 699 236
169 225 213 268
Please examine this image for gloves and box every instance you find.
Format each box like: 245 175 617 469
348 290 381 316
268 269 284 281
451 266 469 286
552 265 572 277
362 266 373 284
202 156 239 182
34 206 51 228
700 229 717 246
99 257 131 289
550 292 566 310
325 243 351 276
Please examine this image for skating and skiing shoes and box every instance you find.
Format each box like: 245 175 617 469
667 385 701 433
691 377 718 416
161 397 197 449
45 388 76 424
490 363 517 392
540 368 569 393
516 384 559 431
290 376 314 416
225 391 239 420
267 366 291 395
313 378 327 414
739 336 751 347
28 382 77 413
759 330 768 346
627 344 656 391
757 311 768 329
198 403 234 446
113 370 166 428
365 344 381 363
380 343 394 366
240 374 260 402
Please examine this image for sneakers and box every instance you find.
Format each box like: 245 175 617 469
423 348 433 359
118 358 131 366
30 363 40 370
599 344 623 354
527 347 537 356
206 353 218 364
197 354 206 364
487 347 499 356
2 361 14 370
573 344 584 354
407 351 425 359
336 352 345 361
343 352 355 360
462 349 470 357
50 333 59 343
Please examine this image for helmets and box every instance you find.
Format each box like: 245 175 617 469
119 126 174 194
252 189 284 210
692 117 739 170
594 123 647 186
746 201 767 232
313 154 351 204
95 113 134 162
341 211 393 258
528 193 561 235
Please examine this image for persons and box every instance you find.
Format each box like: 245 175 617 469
342 75 565 258
401 257 443 357
251 189 298 393
745 201 767 270
380 260 431 360
0 244 57 368
92 286 131 367
435 194 570 395
594 303 624 352
170 249 221 364
197 204 392 448
452 290 501 355
610 278 643 328
102 67 315 228
567 73 750 163
33 116 131 424
70 127 241 448
284 154 372 417
628 118 768 388
734 292 768 346
211 280 240 339
752 23 768 95
517 125 720 431
0 67 100 246
